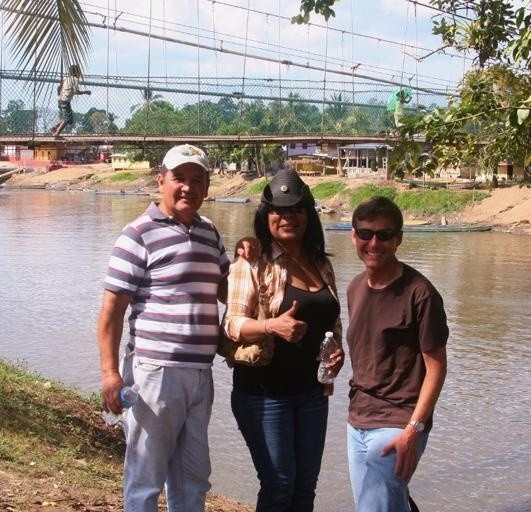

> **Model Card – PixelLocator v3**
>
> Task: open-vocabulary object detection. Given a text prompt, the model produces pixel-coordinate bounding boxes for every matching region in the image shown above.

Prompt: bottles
[104,383,142,427]
[316,331,336,386]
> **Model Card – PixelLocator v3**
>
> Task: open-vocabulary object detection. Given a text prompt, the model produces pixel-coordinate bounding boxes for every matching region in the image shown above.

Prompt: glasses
[354,224,399,241]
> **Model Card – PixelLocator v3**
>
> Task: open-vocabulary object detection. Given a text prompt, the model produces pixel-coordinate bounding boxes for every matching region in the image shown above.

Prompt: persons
[394,91,412,128]
[220,168,347,512]
[344,195,450,511]
[97,145,262,512]
[51,65,92,138]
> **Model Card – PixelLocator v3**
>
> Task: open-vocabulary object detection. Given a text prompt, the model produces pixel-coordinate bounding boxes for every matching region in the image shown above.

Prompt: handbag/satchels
[213,257,272,370]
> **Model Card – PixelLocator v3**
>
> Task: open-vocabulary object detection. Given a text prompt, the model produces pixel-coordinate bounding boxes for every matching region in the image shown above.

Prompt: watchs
[408,420,426,434]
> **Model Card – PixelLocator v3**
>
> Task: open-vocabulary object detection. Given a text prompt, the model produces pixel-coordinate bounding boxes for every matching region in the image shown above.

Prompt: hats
[161,143,210,173]
[261,168,315,209]
